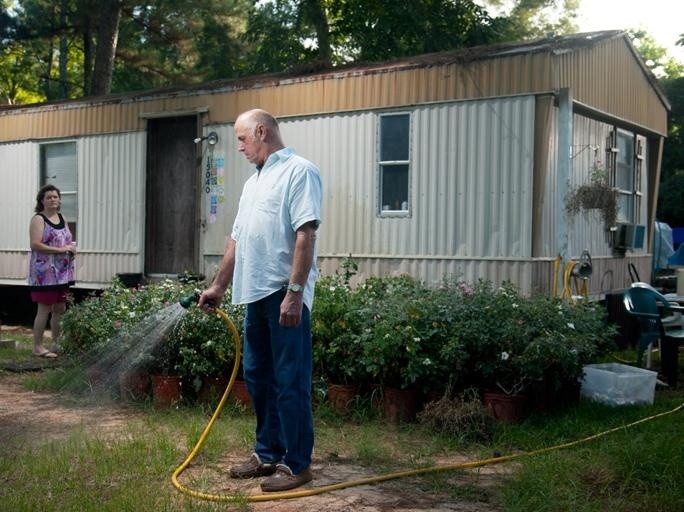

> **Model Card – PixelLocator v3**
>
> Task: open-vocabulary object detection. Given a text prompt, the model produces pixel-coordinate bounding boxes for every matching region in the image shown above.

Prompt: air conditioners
[613,220,646,250]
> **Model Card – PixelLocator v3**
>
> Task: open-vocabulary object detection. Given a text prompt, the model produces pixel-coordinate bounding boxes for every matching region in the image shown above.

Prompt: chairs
[622,281,684,388]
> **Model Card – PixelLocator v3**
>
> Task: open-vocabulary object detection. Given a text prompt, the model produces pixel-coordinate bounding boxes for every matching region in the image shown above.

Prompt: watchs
[281,282,304,295]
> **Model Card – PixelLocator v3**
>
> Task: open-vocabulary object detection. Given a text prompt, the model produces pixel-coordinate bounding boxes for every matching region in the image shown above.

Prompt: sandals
[259,463,313,492]
[230,452,275,479]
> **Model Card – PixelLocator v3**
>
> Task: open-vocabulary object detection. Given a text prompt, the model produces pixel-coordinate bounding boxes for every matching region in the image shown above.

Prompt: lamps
[193,131,218,146]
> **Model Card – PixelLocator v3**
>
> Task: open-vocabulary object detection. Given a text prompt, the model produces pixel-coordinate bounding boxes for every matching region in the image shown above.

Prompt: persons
[26,181,80,361]
[192,107,327,495]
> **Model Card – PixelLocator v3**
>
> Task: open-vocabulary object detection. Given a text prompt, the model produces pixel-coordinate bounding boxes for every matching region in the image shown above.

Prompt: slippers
[32,350,58,358]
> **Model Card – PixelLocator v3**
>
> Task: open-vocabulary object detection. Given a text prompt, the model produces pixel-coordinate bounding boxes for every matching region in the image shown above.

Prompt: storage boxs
[580,363,657,413]
